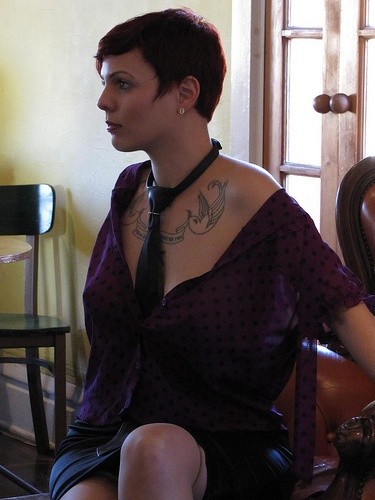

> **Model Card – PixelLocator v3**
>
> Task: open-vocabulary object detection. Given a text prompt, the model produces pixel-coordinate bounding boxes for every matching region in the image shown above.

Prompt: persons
[44,8,374,500]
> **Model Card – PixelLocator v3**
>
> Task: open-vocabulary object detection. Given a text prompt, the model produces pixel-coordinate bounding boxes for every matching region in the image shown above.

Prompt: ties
[125,137,225,342]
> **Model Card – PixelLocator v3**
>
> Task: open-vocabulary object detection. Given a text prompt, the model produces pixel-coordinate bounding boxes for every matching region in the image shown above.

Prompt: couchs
[273,343,375,500]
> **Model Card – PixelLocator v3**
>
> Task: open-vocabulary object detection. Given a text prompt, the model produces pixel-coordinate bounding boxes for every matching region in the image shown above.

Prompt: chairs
[0,183,70,459]
[335,158,375,295]
